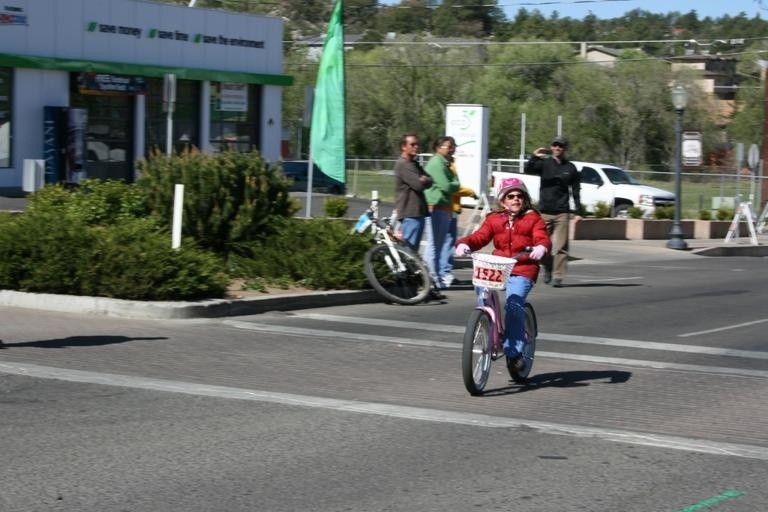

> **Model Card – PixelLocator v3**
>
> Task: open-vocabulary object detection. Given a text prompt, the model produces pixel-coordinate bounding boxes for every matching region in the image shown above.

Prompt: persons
[439,136,479,286]
[523,136,583,287]
[393,131,434,250]
[455,178,552,371]
[424,136,460,288]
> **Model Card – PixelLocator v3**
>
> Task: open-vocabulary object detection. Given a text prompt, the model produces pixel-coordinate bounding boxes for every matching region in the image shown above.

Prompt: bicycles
[461,248,539,396]
[347,196,450,305]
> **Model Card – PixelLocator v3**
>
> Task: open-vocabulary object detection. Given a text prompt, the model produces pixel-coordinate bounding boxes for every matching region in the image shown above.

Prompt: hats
[551,135,567,145]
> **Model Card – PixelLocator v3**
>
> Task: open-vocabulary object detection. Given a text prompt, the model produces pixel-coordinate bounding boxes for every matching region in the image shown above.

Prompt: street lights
[666,82,689,250]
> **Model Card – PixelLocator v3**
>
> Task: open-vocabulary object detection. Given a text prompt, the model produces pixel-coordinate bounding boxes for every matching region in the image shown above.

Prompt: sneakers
[444,275,458,286]
[551,278,561,287]
[542,267,554,282]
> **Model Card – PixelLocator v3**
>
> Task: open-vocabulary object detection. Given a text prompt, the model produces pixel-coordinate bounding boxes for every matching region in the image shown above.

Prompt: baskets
[473,252,521,290]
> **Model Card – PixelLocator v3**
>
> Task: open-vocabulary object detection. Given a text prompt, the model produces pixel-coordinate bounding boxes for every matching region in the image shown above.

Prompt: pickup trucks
[489,155,676,219]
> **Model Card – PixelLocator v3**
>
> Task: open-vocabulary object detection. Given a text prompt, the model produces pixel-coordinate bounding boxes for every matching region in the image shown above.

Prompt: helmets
[497,178,528,199]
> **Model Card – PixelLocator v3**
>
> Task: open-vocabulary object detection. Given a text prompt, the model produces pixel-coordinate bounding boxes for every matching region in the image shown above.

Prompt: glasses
[507,194,524,199]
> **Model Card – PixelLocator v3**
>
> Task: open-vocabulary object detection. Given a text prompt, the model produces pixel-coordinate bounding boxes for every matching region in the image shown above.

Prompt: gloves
[456,244,470,256]
[526,245,545,260]
